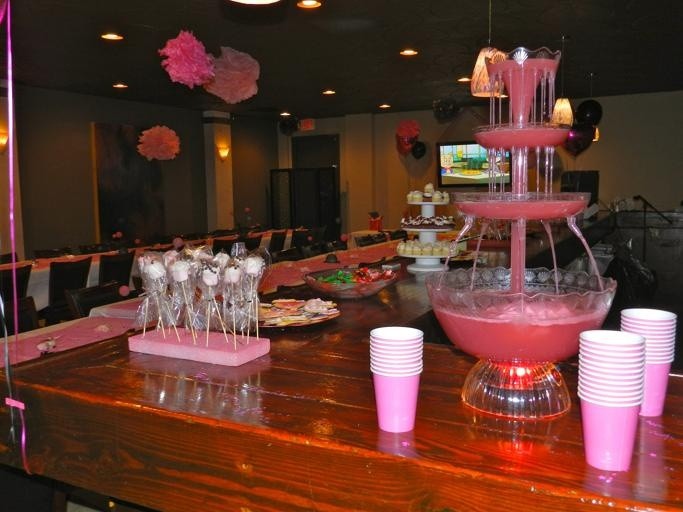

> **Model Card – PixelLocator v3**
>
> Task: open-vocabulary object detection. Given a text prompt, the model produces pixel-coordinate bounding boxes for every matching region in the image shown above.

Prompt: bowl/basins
[304,266,398,298]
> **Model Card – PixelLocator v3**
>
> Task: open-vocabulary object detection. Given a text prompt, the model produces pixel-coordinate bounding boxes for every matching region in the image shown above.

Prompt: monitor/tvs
[435,140,512,188]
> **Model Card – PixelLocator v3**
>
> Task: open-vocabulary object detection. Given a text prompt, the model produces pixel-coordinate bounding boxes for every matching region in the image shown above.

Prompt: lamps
[551,32,599,143]
[471,0,508,98]
[217,146,229,162]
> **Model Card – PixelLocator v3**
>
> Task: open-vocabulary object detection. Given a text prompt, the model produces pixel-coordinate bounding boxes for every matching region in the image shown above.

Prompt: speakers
[560,170,599,220]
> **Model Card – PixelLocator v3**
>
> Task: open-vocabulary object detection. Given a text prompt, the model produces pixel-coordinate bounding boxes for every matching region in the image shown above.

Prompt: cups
[618,307,678,416]
[370,326,424,432]
[577,329,646,471]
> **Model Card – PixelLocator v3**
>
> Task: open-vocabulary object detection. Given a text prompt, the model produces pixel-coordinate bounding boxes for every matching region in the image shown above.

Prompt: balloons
[564,123,594,156]
[276,115,297,137]
[431,96,456,122]
[394,120,425,160]
[575,100,603,127]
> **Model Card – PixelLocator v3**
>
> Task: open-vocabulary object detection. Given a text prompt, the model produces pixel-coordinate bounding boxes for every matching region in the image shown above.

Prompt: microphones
[632,194,647,205]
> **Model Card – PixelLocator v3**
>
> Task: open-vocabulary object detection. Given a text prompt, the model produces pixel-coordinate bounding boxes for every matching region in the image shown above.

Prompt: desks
[0,316,157,369]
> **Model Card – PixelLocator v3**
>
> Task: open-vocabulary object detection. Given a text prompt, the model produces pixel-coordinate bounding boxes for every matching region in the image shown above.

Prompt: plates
[254,299,340,328]
